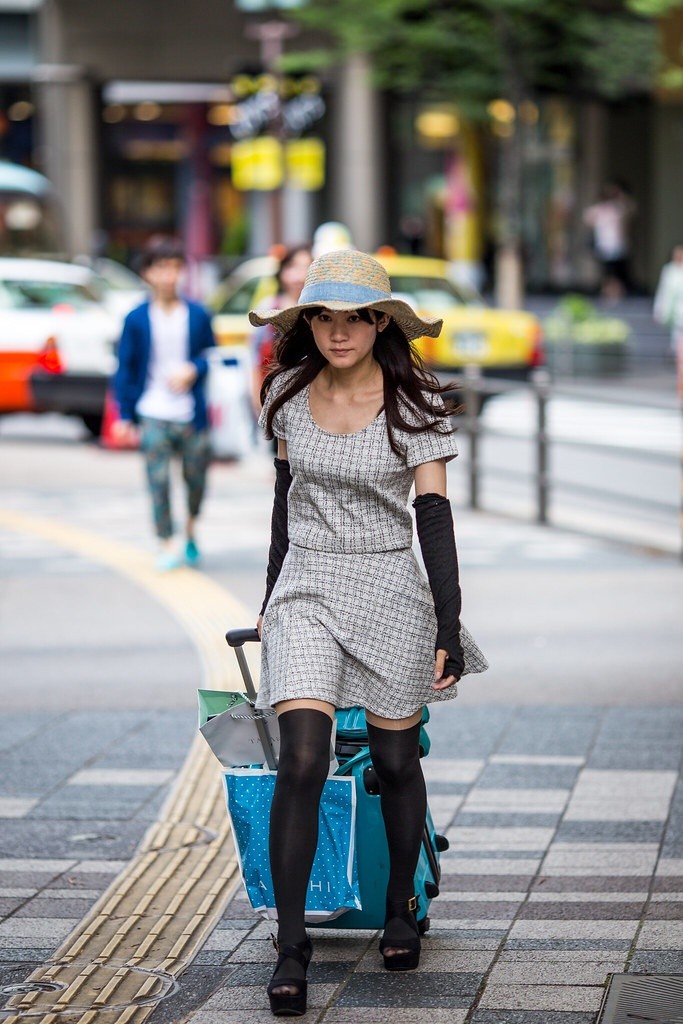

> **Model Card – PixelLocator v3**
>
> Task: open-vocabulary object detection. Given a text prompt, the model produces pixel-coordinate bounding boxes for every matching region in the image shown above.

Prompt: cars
[0,258,121,435]
[205,245,541,374]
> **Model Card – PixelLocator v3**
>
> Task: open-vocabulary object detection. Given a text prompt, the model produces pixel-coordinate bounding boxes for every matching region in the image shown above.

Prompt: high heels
[379,894,421,971]
[267,933,313,1016]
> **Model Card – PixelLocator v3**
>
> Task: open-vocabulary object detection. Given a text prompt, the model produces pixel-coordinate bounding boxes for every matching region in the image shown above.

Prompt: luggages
[226,624,450,934]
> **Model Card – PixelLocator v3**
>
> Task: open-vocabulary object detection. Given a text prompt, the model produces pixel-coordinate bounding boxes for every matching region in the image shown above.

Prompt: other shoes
[160,545,174,569]
[183,532,199,560]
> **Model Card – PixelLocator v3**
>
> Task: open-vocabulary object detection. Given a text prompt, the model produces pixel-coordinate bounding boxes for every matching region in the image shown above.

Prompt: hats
[248,251,444,342]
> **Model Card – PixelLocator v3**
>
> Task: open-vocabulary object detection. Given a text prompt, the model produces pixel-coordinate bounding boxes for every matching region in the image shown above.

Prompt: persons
[576,173,641,299]
[250,250,316,420]
[107,240,222,568]
[244,251,493,1019]
[650,241,683,401]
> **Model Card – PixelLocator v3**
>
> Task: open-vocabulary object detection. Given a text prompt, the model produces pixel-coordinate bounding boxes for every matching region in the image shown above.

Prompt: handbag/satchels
[201,677,301,774]
[224,761,363,924]
